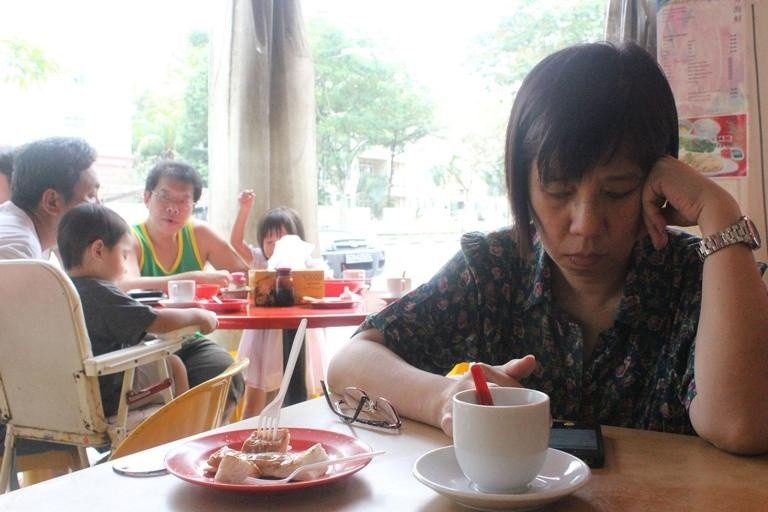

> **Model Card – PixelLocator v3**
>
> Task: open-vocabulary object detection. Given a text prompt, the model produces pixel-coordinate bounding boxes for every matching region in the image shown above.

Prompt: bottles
[228,273,247,290]
[275,268,295,305]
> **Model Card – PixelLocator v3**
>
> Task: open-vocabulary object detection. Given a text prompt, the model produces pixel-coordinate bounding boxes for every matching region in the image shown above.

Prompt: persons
[1,153,12,201]
[121,160,257,428]
[2,136,99,269]
[229,185,310,422]
[56,206,222,419]
[323,40,768,460]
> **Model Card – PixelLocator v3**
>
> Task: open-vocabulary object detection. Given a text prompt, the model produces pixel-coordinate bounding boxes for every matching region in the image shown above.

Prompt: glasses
[319,379,402,430]
[151,190,198,211]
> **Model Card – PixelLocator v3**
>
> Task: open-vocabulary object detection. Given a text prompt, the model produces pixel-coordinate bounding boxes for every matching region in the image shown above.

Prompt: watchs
[695,210,762,262]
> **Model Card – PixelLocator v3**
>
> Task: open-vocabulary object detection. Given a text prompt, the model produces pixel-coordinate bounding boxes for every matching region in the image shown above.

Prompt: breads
[208,429,331,484]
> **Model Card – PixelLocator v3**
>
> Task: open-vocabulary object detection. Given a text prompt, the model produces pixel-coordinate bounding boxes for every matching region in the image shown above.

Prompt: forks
[256,316,308,440]
[244,450,385,485]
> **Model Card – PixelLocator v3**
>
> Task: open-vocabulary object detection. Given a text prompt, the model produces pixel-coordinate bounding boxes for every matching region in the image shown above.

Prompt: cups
[387,278,413,296]
[694,119,721,138]
[220,288,252,304]
[167,280,196,301]
[343,268,365,287]
[452,387,551,492]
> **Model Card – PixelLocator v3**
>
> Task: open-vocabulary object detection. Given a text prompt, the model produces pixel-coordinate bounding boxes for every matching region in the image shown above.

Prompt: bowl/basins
[194,284,220,300]
[323,279,362,297]
[678,119,693,137]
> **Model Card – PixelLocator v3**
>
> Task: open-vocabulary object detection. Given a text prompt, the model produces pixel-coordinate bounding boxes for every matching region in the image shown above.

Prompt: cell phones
[548,421,604,468]
[128,289,163,298]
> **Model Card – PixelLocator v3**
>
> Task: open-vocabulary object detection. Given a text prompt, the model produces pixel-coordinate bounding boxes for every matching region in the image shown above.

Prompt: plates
[381,297,397,303]
[679,139,721,156]
[310,295,353,309]
[410,444,590,512]
[159,299,198,308]
[679,151,739,178]
[162,429,374,492]
[198,299,249,310]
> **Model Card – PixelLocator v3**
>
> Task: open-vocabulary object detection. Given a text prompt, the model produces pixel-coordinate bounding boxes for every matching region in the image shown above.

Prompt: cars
[317,237,388,284]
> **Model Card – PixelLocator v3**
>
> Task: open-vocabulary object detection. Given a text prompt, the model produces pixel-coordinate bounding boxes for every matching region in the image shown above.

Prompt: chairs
[107,356,251,462]
[1,258,206,498]
